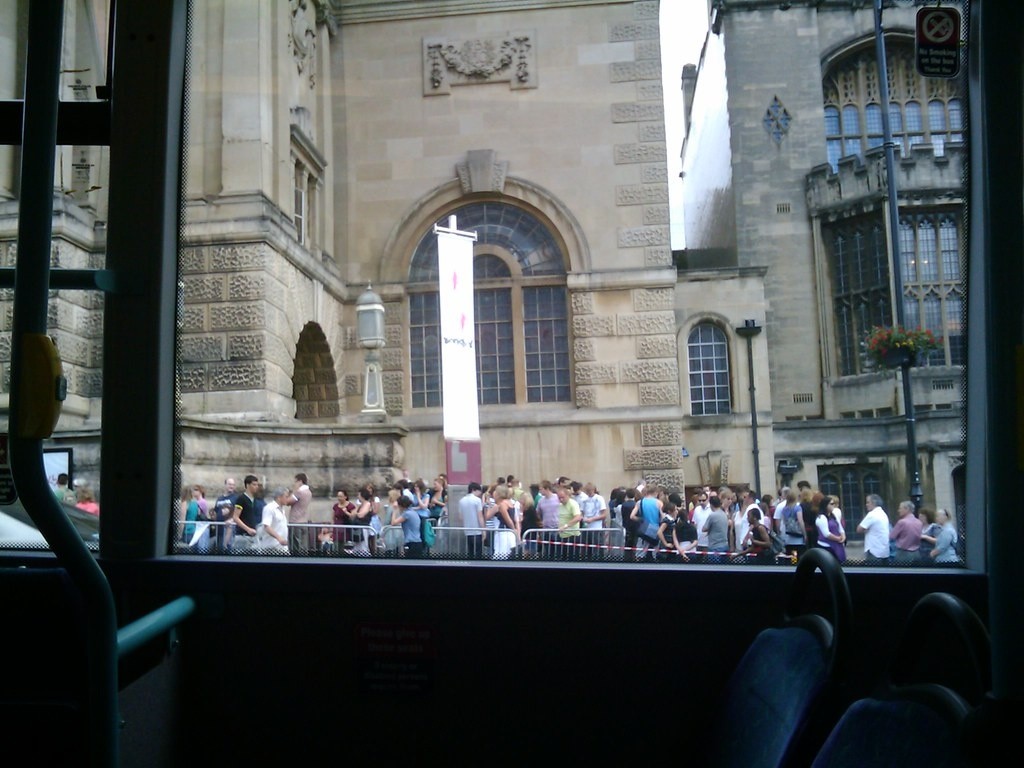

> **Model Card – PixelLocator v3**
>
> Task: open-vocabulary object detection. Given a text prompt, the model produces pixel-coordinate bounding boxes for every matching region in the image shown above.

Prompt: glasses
[698,499,706,502]
[830,503,834,505]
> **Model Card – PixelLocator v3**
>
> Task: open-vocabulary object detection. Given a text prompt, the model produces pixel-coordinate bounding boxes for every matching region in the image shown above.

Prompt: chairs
[812,593,1024,768]
[719,548,855,768]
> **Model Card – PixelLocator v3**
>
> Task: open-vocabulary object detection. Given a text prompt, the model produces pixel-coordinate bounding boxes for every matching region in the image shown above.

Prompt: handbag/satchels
[638,520,659,546]
[419,514,436,547]
[197,505,216,538]
[757,524,785,557]
[349,518,364,542]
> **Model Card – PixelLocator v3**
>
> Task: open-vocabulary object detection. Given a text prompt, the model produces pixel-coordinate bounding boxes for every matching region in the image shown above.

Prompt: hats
[73,478,86,486]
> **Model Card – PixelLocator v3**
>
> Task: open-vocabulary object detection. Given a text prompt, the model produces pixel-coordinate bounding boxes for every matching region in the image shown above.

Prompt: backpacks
[950,532,964,555]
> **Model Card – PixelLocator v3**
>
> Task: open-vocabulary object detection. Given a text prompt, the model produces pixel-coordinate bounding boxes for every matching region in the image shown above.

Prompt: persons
[53,474,99,516]
[174,472,958,563]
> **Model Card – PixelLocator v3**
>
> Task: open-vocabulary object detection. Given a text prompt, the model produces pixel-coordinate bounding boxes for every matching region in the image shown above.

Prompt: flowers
[864,326,944,380]
